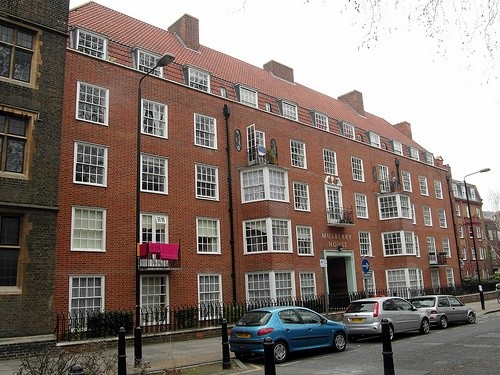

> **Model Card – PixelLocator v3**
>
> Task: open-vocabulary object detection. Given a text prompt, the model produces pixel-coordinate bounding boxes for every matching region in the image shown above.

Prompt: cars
[410,294,477,328]
[342,297,432,343]
[229,307,348,364]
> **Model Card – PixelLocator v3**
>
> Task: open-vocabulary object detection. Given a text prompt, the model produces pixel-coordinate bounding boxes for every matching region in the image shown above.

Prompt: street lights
[133,53,176,366]
[464,167,492,309]
